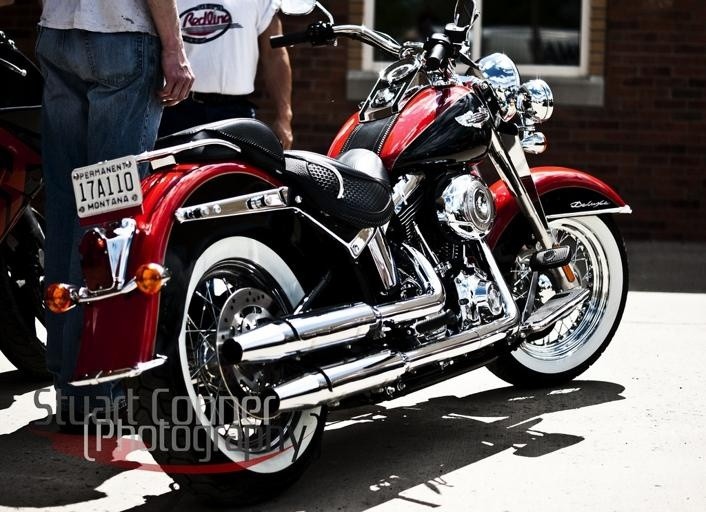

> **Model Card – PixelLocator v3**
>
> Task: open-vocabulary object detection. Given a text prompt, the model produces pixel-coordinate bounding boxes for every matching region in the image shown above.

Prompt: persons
[34,0,195,436]
[153,0,294,153]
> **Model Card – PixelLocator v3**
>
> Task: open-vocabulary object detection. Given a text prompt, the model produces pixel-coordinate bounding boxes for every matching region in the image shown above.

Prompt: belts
[179,91,250,107]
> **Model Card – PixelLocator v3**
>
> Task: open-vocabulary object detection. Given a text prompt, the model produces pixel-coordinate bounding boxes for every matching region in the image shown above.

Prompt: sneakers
[55,390,143,430]
